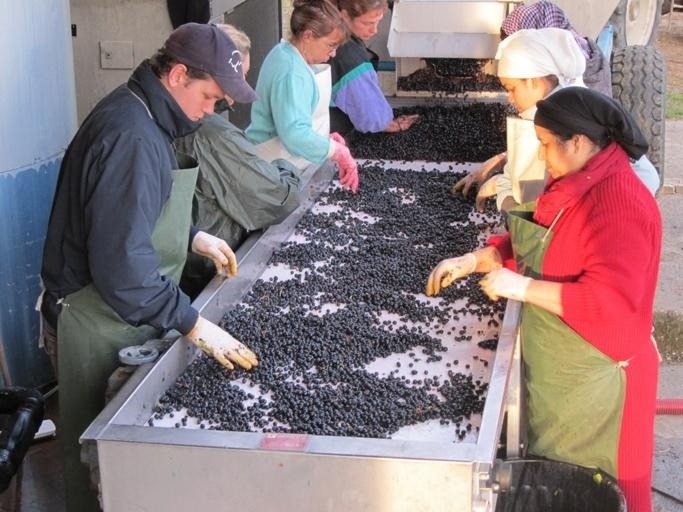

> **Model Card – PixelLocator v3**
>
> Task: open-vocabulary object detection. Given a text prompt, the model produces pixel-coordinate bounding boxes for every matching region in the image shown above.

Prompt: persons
[326,1,419,138]
[32,20,260,511]
[423,86,663,511]
[473,28,660,218]
[451,1,613,199]
[245,1,361,197]
[165,20,303,303]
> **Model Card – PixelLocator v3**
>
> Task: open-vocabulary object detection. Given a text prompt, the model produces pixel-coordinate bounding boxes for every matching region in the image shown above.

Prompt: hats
[158,21,258,105]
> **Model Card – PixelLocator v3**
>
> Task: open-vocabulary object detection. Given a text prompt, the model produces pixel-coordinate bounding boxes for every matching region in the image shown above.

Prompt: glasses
[314,34,341,52]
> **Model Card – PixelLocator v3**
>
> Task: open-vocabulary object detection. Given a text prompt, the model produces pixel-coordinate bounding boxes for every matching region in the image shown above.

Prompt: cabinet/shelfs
[75,94,520,510]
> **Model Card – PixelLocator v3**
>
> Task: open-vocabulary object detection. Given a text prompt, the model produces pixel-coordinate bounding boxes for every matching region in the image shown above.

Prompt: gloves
[422,251,478,297]
[478,265,533,303]
[190,229,238,280]
[451,151,506,197]
[327,130,361,194]
[187,317,259,372]
[475,171,503,212]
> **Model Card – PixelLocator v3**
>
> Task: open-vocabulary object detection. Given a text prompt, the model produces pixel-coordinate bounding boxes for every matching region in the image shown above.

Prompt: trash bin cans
[495,459,627,512]
